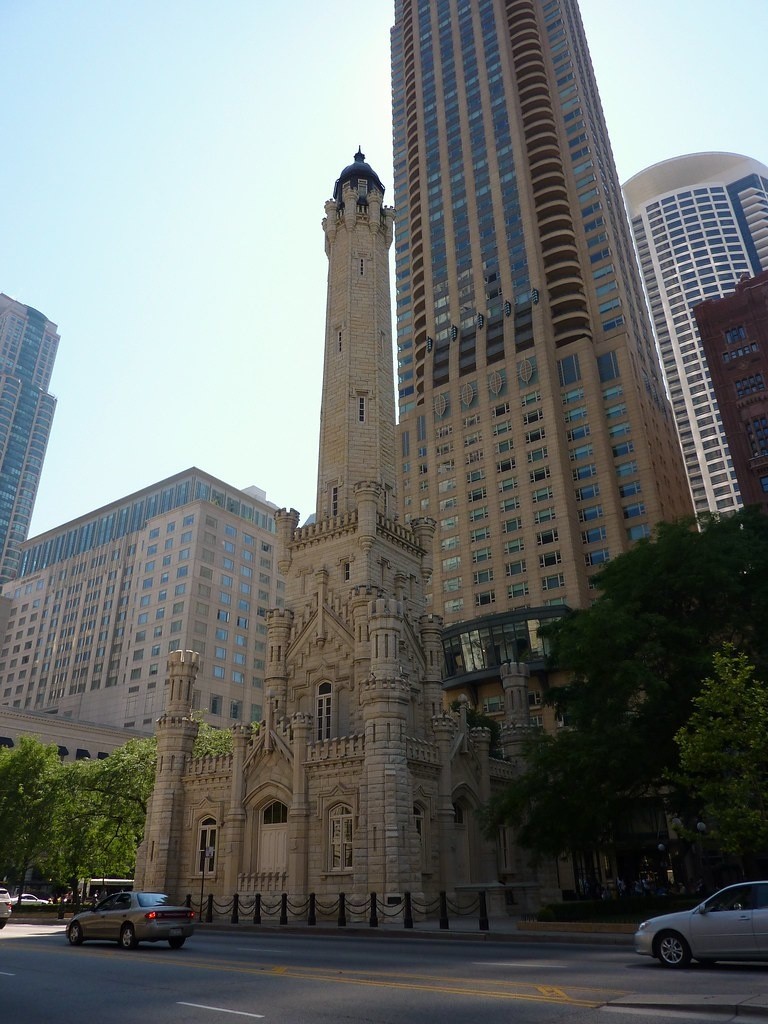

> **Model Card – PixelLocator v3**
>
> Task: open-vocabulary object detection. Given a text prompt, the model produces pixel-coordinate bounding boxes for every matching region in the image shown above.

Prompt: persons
[634,881,640,895]
[99,888,107,899]
[696,878,704,893]
[93,892,98,902]
[498,876,517,905]
[644,873,656,895]
[63,891,73,903]
[79,888,83,898]
[617,877,622,889]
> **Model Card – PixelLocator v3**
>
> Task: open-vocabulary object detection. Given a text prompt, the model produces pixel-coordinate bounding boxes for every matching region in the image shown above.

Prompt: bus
[87,878,134,898]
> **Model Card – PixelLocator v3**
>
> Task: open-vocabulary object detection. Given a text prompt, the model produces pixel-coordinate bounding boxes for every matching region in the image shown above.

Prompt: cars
[634,880,768,969]
[0,887,13,930]
[9,893,50,905]
[65,891,196,951]
